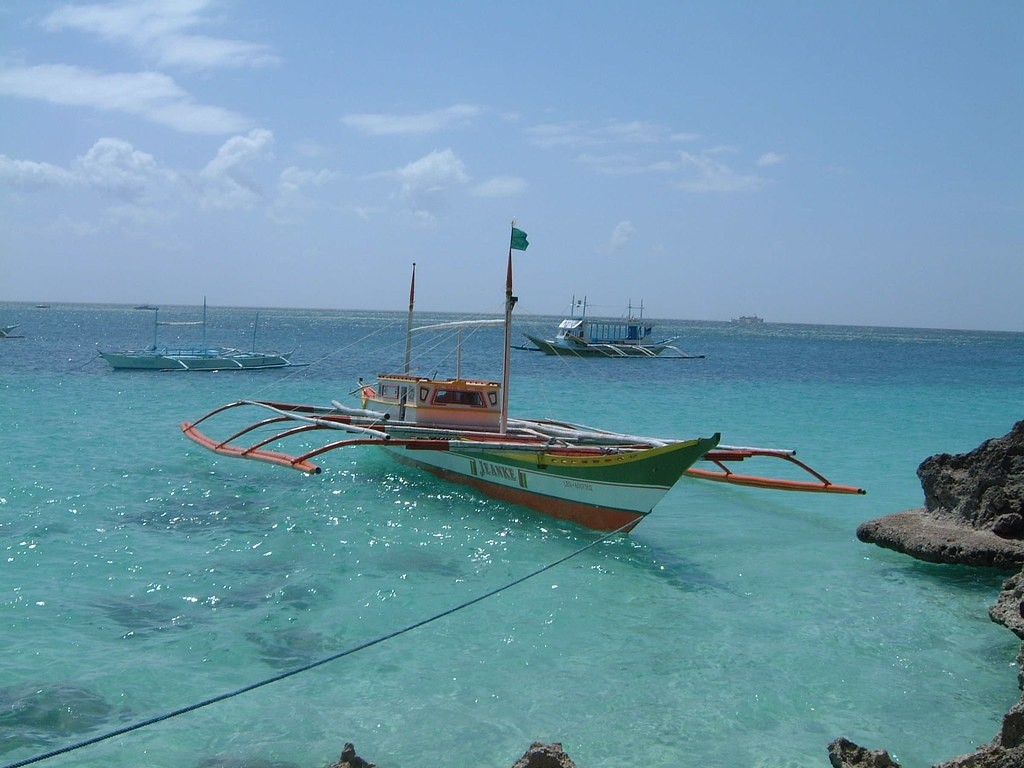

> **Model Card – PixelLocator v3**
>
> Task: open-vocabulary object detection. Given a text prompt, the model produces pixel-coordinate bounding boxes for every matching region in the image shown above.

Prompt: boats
[730,315,765,326]
[131,302,162,310]
[36,303,53,310]
[152,316,206,327]
[523,294,681,355]
[96,295,296,370]
[1,322,26,340]
[371,218,722,538]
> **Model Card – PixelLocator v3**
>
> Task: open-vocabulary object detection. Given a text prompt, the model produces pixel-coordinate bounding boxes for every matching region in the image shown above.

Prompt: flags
[510,228,529,250]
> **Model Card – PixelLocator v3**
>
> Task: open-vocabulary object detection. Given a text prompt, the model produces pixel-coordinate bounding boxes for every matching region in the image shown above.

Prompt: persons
[461,393,475,403]
